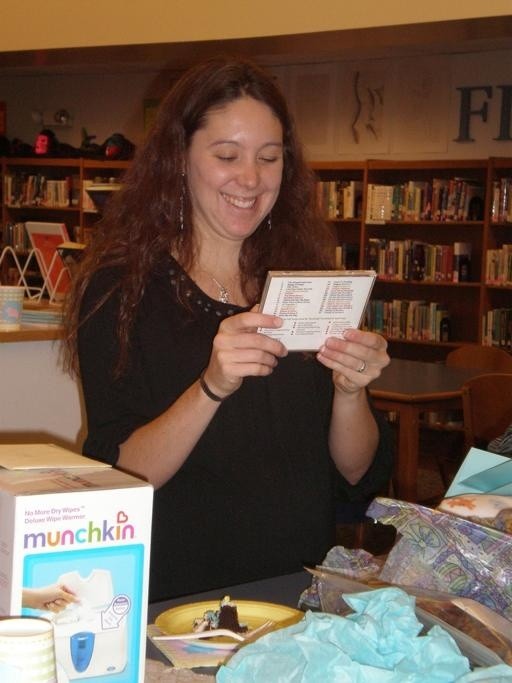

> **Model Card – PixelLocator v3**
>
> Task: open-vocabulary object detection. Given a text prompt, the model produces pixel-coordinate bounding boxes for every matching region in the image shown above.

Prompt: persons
[51,58,391,605]
[21,582,81,612]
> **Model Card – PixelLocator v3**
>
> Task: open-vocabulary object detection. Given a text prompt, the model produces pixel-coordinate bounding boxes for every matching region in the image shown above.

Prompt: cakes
[193,595,247,640]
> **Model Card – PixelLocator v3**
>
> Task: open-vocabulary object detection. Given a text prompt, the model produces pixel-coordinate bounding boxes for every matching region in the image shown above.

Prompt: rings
[356,362,366,373]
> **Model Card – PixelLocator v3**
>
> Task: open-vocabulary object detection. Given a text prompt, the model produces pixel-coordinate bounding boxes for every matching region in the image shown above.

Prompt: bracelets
[200,367,227,402]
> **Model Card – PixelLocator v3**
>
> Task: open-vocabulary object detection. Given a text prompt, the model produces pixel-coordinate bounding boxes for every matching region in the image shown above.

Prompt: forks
[152,618,276,642]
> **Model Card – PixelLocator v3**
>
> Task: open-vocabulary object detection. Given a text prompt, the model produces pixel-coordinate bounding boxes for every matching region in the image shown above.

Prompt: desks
[145,571,321,683]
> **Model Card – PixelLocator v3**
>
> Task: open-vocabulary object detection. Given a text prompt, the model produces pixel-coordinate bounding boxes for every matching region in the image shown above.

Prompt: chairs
[341,345,512,549]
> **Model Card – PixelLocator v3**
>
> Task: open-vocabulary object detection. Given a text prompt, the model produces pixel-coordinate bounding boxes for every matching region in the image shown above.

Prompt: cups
[3,616,55,683]
[0,286,26,331]
[69,629,96,672]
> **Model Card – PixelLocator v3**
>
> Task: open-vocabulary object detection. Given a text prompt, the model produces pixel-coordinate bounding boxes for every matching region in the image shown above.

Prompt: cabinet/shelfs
[0,155,512,364]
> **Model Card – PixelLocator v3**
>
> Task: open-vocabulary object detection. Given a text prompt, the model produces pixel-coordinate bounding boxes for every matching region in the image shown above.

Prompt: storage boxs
[0,442,154,683]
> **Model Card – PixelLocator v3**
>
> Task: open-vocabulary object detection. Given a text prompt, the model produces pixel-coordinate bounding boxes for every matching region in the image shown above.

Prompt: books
[313,177,484,342]
[486,177,511,346]
[1,172,123,301]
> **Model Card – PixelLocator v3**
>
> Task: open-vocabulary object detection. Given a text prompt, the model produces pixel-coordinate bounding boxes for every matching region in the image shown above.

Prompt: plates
[155,597,306,650]
